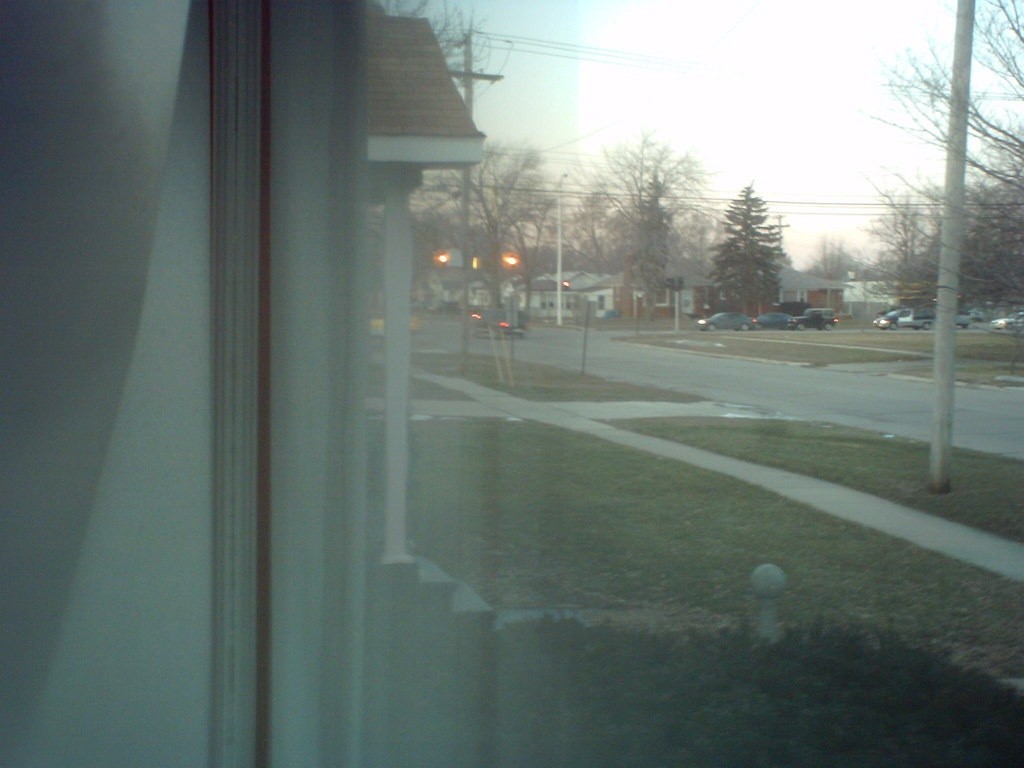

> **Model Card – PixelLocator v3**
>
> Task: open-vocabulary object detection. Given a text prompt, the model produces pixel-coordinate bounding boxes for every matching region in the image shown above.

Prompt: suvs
[794,307,840,330]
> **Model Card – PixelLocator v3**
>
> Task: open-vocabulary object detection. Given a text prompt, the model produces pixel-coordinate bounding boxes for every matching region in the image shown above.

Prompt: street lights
[555,172,565,326]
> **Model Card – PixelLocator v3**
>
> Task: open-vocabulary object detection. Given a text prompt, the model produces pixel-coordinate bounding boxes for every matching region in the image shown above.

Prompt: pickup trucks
[873,308,972,329]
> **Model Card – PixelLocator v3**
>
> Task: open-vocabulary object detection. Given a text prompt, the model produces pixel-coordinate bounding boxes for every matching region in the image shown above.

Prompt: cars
[696,312,757,331]
[756,313,798,331]
[991,311,1024,330]
[596,309,622,320]
